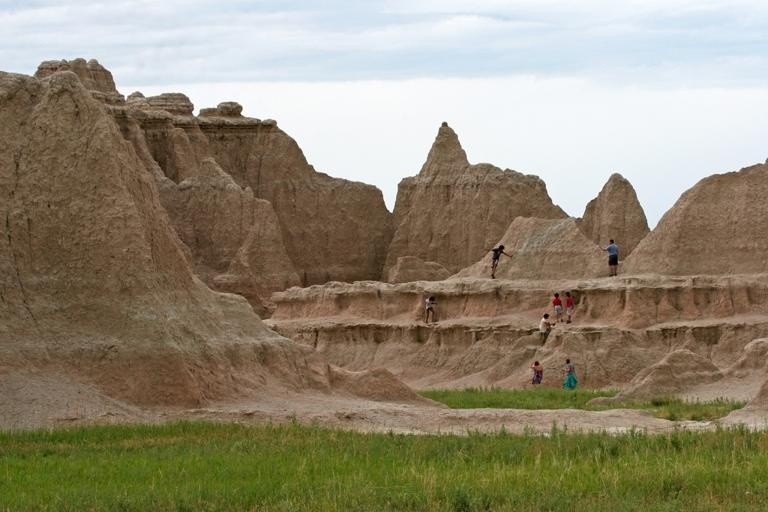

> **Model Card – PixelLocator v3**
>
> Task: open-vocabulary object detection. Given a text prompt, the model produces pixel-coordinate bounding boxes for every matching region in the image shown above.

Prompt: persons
[482,244,512,279]
[530,361,544,385]
[539,313,555,345]
[552,293,563,323]
[565,291,574,324]
[424,295,439,323]
[599,239,619,277]
[561,358,579,391]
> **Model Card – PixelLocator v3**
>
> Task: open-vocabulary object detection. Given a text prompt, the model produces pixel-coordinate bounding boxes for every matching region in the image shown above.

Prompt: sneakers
[609,273,616,276]
